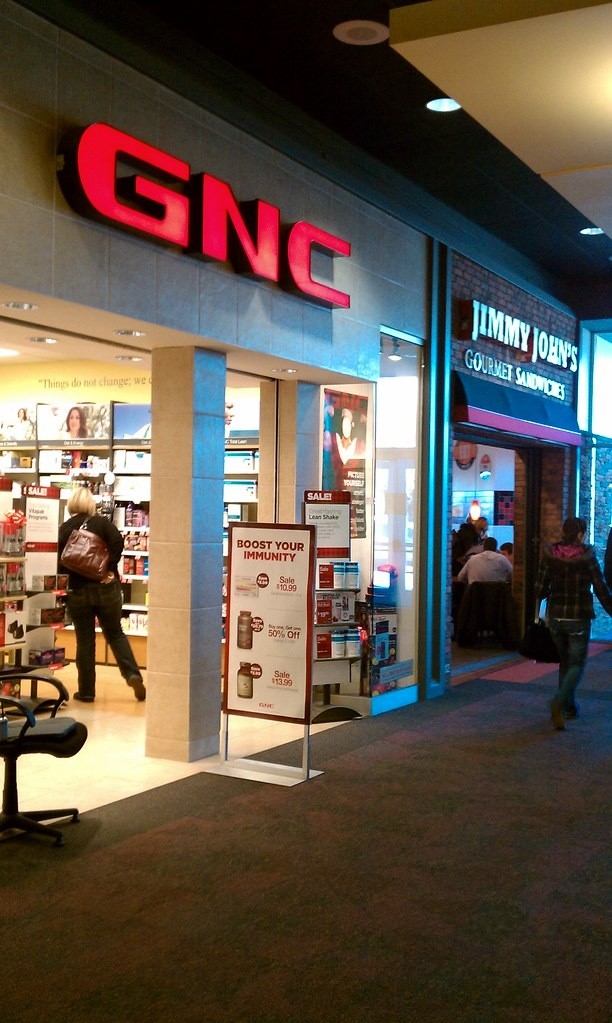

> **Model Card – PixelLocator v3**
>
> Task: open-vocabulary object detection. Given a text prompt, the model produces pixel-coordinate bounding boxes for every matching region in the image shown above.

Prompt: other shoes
[73,691,94,702]
[127,673,146,701]
[549,697,567,730]
[562,687,578,716]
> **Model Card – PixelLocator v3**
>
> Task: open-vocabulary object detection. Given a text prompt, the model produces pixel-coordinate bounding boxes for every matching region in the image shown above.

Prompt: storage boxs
[223,451,254,471]
[224,478,257,499]
[227,504,242,522]
[20,456,33,468]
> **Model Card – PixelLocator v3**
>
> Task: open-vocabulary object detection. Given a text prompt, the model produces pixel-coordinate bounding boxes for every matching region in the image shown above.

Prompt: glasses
[478,526,488,532]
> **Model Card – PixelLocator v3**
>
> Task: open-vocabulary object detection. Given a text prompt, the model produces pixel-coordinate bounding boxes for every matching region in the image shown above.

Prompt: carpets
[480,641,610,684]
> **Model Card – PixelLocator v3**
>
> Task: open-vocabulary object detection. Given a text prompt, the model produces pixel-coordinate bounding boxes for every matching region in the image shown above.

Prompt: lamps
[470,458,481,520]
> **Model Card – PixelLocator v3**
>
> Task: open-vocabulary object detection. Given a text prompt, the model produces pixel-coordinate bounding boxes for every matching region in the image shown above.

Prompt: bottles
[73,480,111,496]
[237,662,253,699]
[237,611,253,650]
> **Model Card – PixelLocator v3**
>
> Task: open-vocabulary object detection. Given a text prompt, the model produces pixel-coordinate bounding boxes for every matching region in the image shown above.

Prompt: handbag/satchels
[518,616,546,659]
[60,515,111,581]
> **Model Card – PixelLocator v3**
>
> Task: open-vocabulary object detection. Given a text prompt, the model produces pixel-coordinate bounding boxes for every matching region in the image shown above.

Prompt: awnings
[450,371,582,448]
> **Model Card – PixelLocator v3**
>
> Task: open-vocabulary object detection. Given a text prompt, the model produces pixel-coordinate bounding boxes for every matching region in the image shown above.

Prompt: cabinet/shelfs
[0,447,259,721]
[309,589,364,724]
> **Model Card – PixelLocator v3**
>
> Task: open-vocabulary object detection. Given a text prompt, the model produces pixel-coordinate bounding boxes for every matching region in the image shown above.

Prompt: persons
[60,406,89,439]
[500,542,513,562]
[458,537,519,650]
[57,486,146,703]
[452,517,489,577]
[604,528,612,594]
[534,518,612,730]
[225,399,235,424]
[10,408,33,440]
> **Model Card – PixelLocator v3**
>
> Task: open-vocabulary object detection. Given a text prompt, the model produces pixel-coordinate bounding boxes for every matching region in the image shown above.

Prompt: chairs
[456,580,516,650]
[0,670,88,848]
